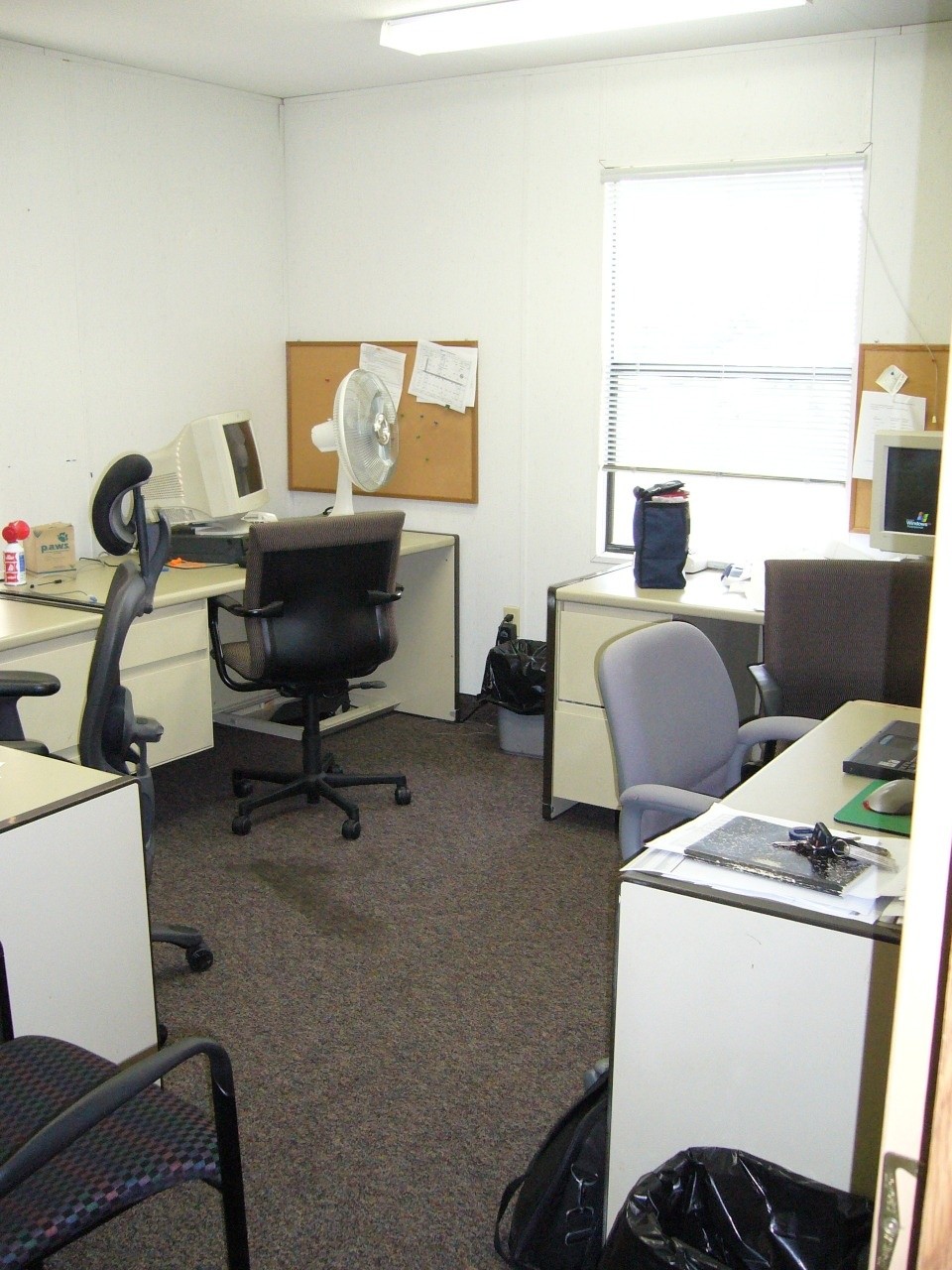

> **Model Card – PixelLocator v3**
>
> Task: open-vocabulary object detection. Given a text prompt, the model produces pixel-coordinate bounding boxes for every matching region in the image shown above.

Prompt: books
[684,815,891,896]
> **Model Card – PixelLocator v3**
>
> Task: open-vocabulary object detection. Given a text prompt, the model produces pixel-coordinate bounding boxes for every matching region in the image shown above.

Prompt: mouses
[862,778,915,816]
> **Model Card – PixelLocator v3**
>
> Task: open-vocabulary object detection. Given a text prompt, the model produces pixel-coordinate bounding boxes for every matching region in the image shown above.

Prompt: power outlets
[504,606,520,637]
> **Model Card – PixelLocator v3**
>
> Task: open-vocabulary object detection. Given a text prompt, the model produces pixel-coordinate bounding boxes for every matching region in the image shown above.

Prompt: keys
[772,823,861,856]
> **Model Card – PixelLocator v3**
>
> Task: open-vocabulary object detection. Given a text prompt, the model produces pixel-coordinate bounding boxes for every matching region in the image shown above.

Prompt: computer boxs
[169,523,252,565]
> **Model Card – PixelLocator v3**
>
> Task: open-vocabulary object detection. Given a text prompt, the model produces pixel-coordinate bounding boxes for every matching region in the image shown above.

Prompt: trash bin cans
[625,1148,871,1270]
[491,639,547,757]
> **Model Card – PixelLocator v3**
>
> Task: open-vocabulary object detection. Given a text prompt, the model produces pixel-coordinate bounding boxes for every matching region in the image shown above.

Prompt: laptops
[841,719,920,779]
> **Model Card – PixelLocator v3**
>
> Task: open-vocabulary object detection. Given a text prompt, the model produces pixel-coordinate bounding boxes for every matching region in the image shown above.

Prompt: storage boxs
[23,521,77,573]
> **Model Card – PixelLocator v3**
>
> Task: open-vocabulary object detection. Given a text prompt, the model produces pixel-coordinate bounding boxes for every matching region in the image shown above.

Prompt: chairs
[746,560,932,773]
[206,510,414,841]
[0,454,215,1050]
[0,940,251,1270]
[593,621,826,860]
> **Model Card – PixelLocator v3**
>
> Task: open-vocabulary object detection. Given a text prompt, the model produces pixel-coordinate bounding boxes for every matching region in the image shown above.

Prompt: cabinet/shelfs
[543,561,764,820]
[600,700,921,1244]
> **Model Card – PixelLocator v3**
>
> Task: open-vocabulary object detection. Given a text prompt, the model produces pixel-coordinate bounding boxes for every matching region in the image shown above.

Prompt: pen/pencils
[30,580,64,588]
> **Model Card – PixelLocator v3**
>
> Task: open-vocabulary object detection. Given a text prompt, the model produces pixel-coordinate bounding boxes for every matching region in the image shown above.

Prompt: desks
[0,747,164,1089]
[0,530,461,773]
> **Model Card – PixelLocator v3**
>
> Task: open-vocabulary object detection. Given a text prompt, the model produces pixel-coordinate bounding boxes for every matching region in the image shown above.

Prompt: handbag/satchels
[494,1069,609,1270]
[632,481,690,589]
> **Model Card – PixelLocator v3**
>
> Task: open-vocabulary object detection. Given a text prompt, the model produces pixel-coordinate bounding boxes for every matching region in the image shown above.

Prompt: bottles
[652,489,689,500]
[2,541,26,585]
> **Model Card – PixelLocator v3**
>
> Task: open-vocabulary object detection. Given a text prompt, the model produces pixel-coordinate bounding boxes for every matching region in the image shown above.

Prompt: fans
[310,368,400,517]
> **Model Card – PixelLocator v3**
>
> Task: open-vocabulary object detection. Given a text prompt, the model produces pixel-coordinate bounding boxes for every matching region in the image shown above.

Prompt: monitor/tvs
[129,409,269,536]
[869,430,944,555]
